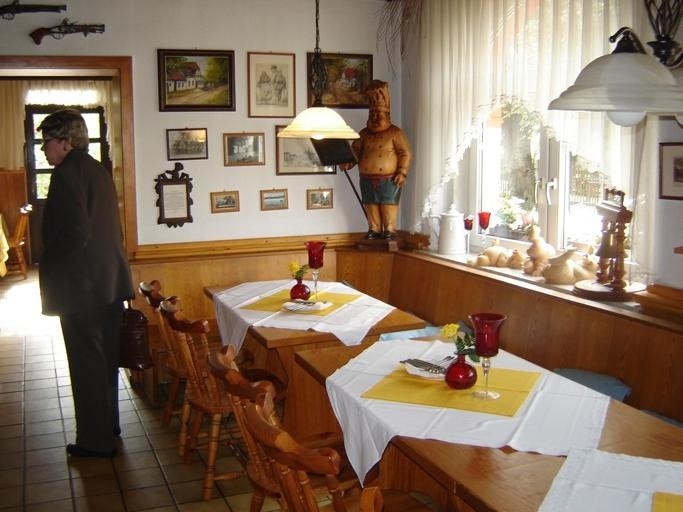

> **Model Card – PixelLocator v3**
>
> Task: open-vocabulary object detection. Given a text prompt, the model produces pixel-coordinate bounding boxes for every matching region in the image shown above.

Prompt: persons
[38,109,135,459]
[327,63,340,103]
[338,79,410,240]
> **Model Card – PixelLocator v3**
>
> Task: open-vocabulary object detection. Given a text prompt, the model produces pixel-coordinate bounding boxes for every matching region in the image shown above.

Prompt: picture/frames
[258,188,288,211]
[305,51,374,110]
[210,189,240,214]
[222,131,266,167]
[305,188,334,211]
[164,127,209,161]
[246,49,298,120]
[657,138,682,202]
[274,123,337,176]
[155,46,237,114]
[152,162,193,228]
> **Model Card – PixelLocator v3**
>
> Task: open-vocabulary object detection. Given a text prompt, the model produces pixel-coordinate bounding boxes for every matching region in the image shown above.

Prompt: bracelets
[398,172,407,178]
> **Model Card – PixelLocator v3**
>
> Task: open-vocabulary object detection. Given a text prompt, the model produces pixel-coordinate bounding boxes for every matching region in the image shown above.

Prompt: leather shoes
[66,440,119,460]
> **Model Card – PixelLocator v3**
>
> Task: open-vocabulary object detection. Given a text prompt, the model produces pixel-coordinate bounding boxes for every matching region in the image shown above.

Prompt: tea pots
[429,203,466,256]
[542,247,577,285]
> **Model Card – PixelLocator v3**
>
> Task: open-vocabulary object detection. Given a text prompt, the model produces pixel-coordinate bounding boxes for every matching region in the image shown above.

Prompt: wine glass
[304,241,328,306]
[469,311,509,402]
[463,218,474,257]
[478,211,491,254]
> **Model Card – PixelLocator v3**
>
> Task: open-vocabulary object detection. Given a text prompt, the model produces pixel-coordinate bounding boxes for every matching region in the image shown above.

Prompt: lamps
[274,0,364,141]
[545,0,683,131]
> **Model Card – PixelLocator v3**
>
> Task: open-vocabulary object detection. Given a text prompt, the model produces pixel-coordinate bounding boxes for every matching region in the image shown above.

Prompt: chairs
[139,280,247,502]
[4,202,33,281]
[207,343,430,512]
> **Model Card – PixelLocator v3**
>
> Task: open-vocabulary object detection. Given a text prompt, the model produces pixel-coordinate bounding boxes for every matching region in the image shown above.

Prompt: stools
[643,407,679,430]
[554,368,630,401]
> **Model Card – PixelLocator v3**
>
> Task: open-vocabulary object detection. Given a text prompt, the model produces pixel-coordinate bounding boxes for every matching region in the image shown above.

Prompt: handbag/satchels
[118,308,154,371]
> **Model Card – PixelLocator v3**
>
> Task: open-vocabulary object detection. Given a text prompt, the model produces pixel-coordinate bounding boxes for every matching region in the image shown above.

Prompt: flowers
[289,259,310,279]
